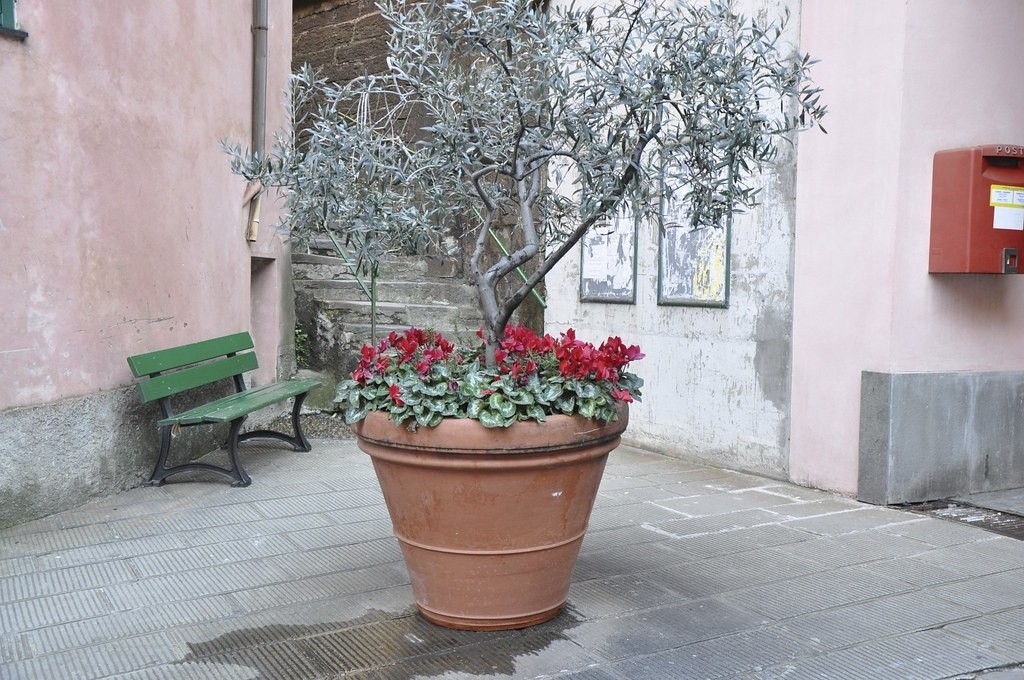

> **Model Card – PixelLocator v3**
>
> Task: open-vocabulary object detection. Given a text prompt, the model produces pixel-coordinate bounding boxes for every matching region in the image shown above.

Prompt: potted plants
[229,0,829,634]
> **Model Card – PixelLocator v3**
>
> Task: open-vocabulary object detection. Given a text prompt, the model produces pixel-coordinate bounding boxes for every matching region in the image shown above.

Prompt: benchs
[126,330,323,489]
